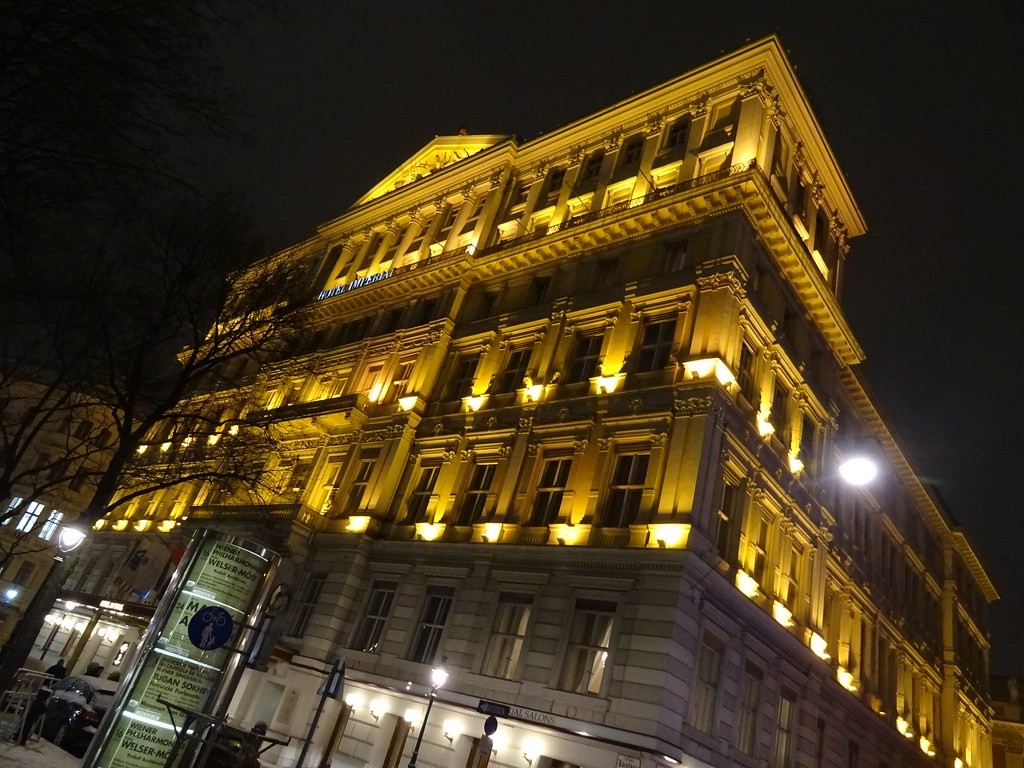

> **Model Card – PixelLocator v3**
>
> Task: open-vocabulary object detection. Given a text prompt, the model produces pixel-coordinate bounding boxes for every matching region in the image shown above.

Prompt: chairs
[0,667,60,746]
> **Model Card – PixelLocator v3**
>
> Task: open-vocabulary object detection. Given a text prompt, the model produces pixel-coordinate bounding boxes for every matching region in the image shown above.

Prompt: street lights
[0,507,96,667]
[405,655,452,768]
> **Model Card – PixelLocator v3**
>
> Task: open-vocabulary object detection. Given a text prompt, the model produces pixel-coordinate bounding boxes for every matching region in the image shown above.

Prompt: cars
[22,674,119,758]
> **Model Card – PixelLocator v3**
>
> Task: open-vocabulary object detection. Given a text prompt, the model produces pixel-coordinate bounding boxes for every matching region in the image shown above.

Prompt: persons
[43,658,67,690]
